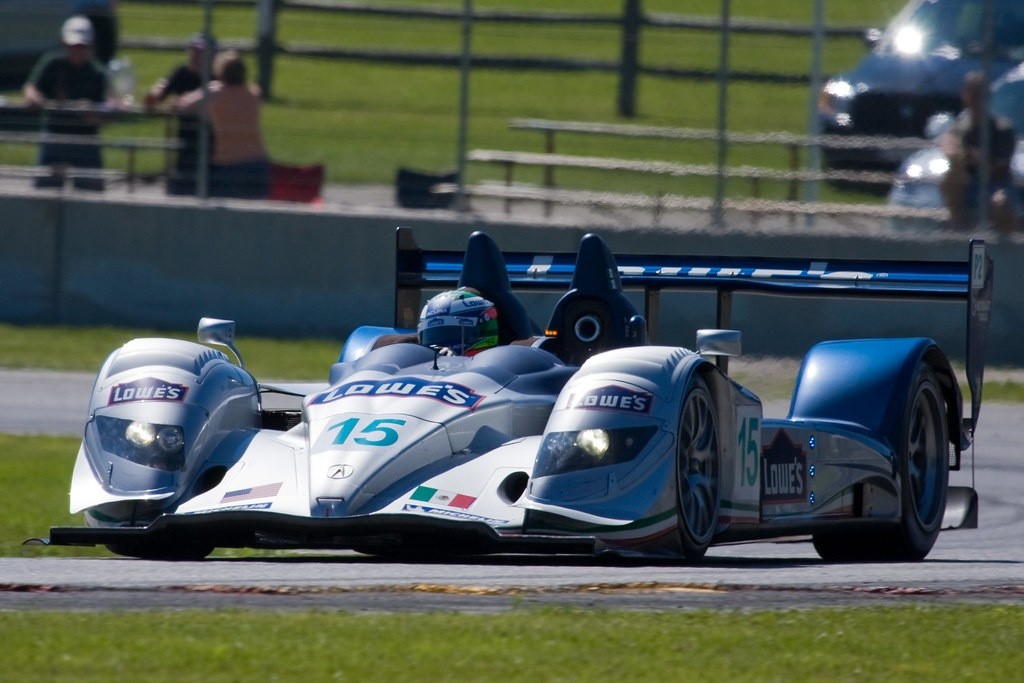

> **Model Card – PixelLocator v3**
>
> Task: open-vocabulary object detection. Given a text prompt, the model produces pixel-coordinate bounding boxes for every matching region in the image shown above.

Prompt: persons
[27,16,125,194]
[141,30,273,198]
[936,74,1018,222]
[419,288,497,357]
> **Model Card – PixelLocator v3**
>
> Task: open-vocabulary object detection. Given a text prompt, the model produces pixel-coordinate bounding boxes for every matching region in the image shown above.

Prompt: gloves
[430,345,457,357]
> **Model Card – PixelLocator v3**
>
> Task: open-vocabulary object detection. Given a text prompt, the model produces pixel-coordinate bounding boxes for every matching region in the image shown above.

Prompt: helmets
[417,289,499,356]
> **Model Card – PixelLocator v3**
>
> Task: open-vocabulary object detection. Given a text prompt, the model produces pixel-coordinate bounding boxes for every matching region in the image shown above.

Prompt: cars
[888,63,1024,228]
[814,1,1022,200]
[1,0,120,89]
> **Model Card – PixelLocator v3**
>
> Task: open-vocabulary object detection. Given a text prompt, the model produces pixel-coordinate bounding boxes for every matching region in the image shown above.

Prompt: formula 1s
[46,218,994,570]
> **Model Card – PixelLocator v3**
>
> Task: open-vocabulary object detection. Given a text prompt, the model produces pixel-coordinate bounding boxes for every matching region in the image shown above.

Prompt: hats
[191,33,218,52]
[61,16,94,46]
